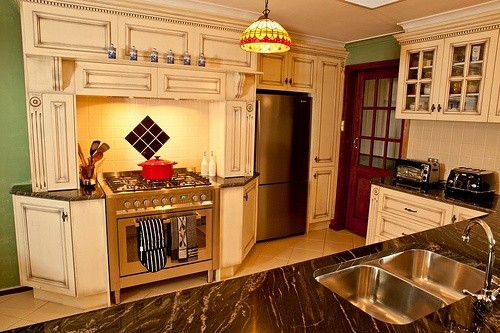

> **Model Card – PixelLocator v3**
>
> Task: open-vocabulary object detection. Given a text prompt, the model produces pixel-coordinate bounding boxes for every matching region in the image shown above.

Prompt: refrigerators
[254,93,313,240]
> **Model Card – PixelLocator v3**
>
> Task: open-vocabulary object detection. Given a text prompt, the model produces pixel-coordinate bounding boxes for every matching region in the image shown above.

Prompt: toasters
[446,166,495,202]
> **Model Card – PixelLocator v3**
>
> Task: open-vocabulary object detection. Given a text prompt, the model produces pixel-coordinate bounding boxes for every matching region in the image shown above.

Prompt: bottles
[150,48,158,64]
[167,49,174,64]
[108,44,116,60]
[198,52,205,68]
[200,151,208,175]
[129,45,137,62]
[183,51,191,66]
[208,150,217,177]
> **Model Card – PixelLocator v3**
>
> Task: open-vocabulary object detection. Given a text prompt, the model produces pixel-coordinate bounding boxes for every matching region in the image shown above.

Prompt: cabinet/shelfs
[395,28,500,124]
[365,184,489,246]
[12,1,351,311]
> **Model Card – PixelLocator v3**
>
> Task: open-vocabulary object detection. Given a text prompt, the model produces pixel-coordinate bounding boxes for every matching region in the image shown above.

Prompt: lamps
[240,0,292,54]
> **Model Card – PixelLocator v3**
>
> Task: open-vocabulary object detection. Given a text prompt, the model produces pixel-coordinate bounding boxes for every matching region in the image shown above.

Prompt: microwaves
[393,159,440,190]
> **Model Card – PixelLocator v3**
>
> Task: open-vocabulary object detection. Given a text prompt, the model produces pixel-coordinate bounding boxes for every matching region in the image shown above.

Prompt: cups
[80,164,97,189]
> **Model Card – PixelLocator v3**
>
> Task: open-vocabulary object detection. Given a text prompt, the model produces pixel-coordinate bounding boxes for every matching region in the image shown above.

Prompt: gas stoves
[100,166,215,218]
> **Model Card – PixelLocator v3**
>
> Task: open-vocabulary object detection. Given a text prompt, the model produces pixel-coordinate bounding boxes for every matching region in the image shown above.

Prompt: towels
[136,218,168,273]
[169,215,198,263]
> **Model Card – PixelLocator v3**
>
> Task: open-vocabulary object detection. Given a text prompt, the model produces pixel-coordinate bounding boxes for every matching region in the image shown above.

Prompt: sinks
[312,259,448,325]
[378,248,496,306]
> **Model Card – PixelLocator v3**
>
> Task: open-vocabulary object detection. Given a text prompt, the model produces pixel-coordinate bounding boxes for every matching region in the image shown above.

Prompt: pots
[136,155,177,183]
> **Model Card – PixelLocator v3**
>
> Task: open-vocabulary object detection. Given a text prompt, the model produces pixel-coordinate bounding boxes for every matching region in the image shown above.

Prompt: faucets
[460,217,496,311]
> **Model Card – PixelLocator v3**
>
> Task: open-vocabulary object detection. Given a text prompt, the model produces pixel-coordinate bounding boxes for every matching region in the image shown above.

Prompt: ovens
[107,206,217,305]
